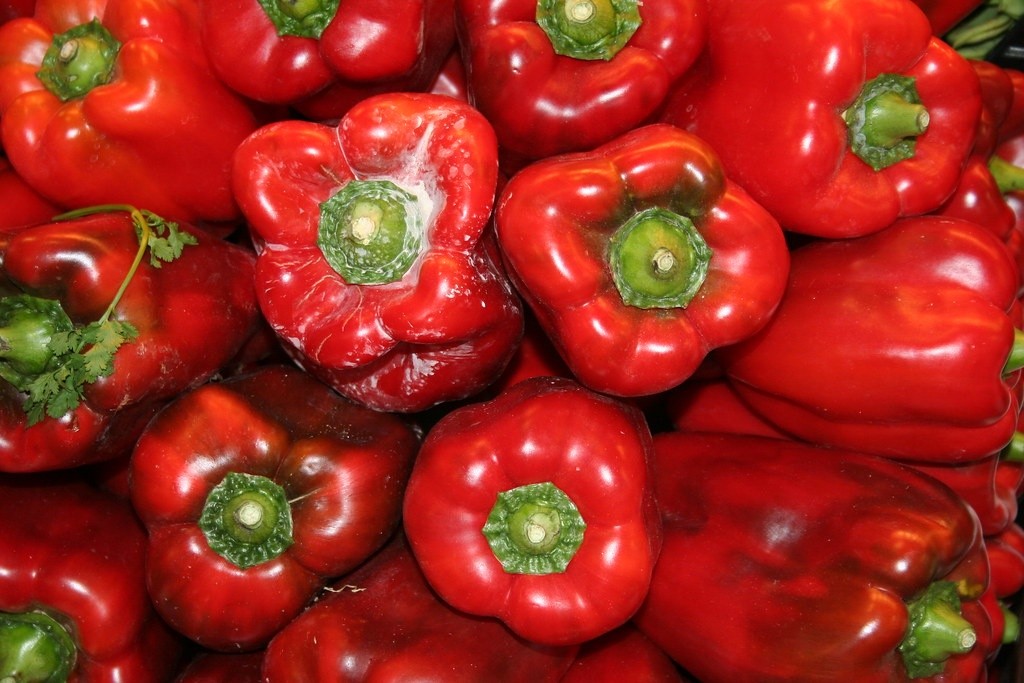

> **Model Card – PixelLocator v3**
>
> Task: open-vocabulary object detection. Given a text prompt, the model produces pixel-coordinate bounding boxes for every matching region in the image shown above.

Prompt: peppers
[1,1,1024,683]
